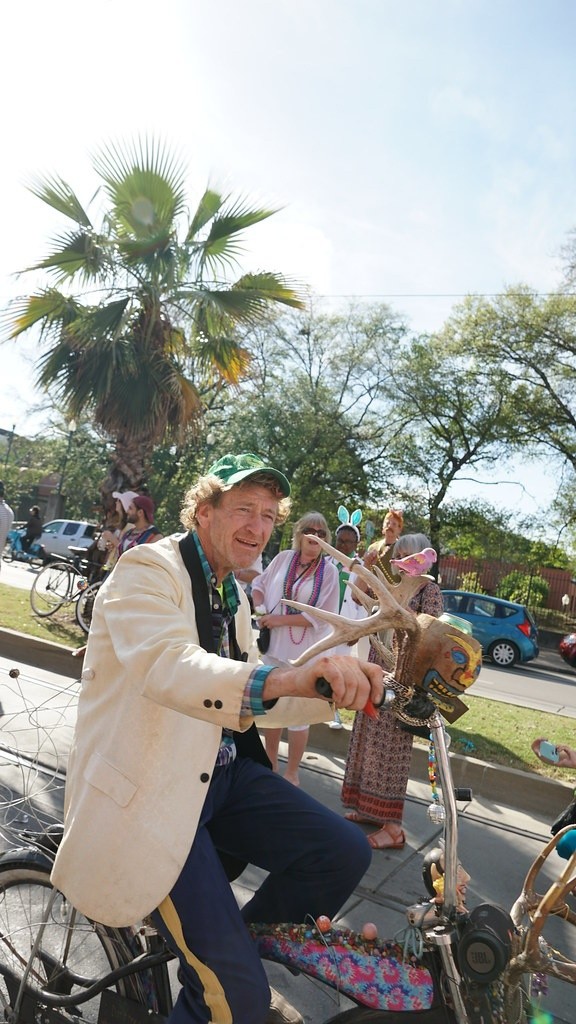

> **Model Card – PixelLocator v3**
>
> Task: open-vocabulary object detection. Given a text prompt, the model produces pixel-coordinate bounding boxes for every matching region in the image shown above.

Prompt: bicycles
[29,546,104,635]
[0,527,46,570]
[0,661,576,1024]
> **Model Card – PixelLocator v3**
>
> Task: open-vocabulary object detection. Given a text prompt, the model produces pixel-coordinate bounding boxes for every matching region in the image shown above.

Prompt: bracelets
[237,569,242,579]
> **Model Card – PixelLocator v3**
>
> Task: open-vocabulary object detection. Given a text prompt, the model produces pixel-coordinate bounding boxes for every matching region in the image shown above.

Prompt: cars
[437,589,541,671]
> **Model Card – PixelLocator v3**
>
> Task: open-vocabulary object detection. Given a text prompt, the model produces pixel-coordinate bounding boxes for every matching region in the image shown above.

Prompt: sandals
[366,825,405,849]
[344,811,384,827]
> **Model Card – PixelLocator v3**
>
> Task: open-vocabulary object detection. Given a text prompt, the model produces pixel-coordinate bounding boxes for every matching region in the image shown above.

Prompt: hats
[208,453,290,498]
[134,496,155,522]
[112,490,139,512]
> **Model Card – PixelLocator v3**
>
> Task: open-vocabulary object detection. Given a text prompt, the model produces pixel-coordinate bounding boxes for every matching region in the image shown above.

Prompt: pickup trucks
[19,518,102,575]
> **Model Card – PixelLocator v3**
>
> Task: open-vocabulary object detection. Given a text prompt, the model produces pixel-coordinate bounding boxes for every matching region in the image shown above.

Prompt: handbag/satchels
[256,625,271,653]
[86,533,108,563]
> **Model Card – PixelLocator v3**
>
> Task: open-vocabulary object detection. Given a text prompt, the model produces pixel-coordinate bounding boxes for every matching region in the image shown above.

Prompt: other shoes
[268,985,305,1024]
[329,710,342,729]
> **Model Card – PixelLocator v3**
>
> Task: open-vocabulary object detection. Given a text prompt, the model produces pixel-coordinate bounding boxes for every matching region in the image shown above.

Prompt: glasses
[337,540,359,546]
[303,527,327,538]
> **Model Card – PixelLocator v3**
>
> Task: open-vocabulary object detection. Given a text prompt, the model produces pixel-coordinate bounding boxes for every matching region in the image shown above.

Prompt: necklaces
[284,551,326,647]
[377,544,397,586]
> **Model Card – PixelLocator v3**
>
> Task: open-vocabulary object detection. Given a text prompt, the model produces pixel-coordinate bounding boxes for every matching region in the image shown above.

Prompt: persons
[94,490,164,586]
[233,552,263,594]
[250,512,340,787]
[361,510,407,651]
[326,507,364,609]
[0,479,14,554]
[17,505,42,552]
[50,455,383,1024]
[341,534,444,848]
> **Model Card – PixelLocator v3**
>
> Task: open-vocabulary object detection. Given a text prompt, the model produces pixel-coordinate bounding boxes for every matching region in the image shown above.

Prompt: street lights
[200,433,216,477]
[3,416,19,467]
[51,418,78,520]
